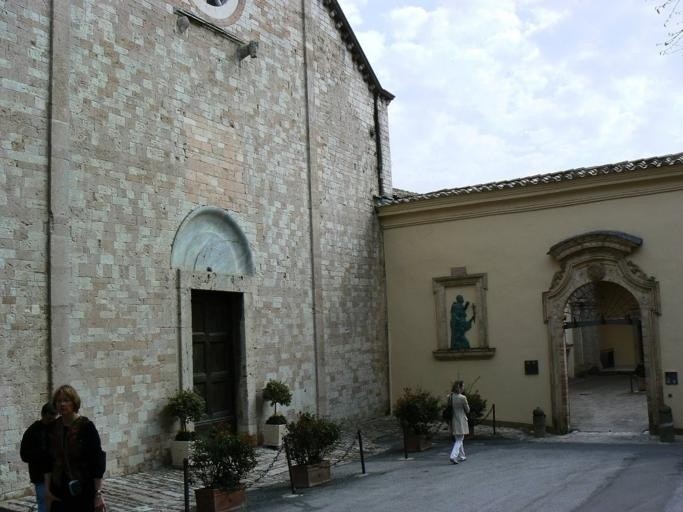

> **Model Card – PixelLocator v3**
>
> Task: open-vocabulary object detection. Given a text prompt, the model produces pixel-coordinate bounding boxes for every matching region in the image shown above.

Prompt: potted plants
[393,384,445,452]
[186,424,260,512]
[453,376,489,440]
[168,390,207,468]
[262,378,292,449]
[284,410,339,489]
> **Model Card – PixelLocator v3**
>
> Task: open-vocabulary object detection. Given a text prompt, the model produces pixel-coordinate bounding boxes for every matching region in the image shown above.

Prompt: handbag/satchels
[443,406,453,420]
[68,480,83,497]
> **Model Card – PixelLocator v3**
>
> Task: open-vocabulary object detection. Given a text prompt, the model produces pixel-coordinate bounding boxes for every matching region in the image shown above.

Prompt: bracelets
[95,487,103,496]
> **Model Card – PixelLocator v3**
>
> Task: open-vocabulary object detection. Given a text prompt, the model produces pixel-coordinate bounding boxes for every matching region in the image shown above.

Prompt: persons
[42,384,108,512]
[446,379,471,465]
[19,402,57,512]
[450,294,473,349]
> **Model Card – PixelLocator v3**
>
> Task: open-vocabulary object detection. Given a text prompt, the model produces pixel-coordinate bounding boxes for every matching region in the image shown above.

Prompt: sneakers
[460,456,466,461]
[450,456,459,464]
